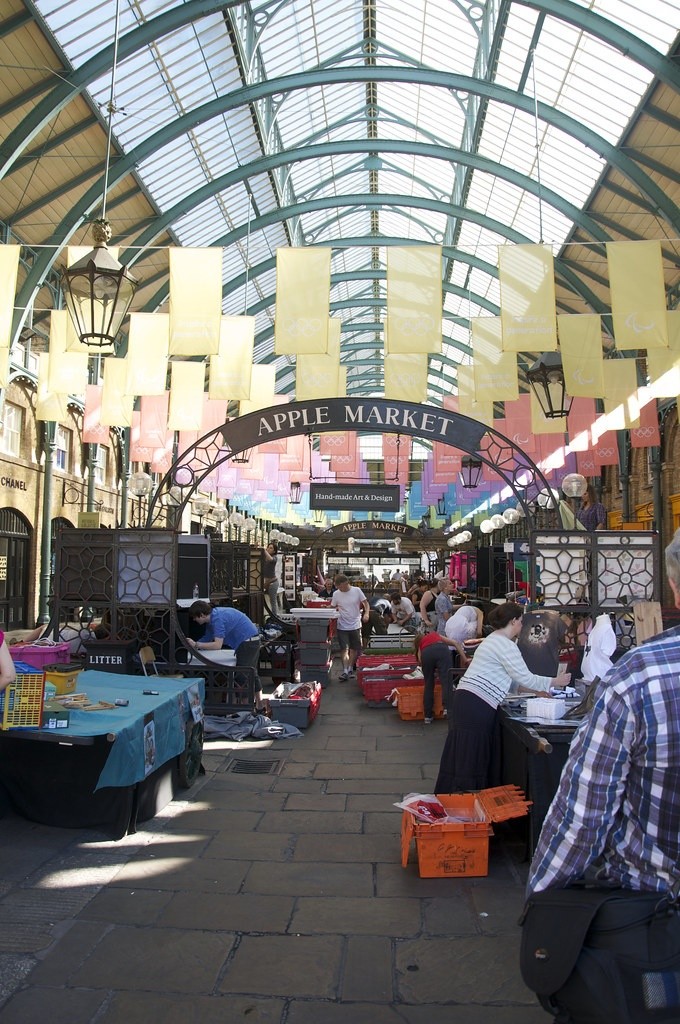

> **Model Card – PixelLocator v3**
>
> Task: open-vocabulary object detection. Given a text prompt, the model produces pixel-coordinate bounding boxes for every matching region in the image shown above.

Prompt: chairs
[138,647,183,678]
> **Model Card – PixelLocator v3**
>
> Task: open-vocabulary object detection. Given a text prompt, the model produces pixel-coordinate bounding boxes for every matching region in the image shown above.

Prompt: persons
[185,600,263,707]
[434,580,454,637]
[445,603,485,689]
[433,602,571,794]
[319,579,335,598]
[525,526,680,1024]
[420,578,442,635]
[381,569,430,612]
[362,593,391,647]
[320,576,369,680]
[0,629,16,692]
[262,543,280,614]
[391,593,416,632]
[418,633,472,724]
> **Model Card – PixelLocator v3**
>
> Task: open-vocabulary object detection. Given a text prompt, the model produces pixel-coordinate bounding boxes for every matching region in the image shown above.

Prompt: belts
[244,636,260,641]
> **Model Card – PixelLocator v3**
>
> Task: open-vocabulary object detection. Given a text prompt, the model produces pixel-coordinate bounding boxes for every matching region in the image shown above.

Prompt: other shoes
[348,670,355,677]
[425,717,433,724]
[339,673,349,680]
[442,708,447,719]
[254,708,265,716]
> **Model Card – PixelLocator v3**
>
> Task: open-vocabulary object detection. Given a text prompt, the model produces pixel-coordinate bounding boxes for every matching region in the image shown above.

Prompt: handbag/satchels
[518,889,680,1024]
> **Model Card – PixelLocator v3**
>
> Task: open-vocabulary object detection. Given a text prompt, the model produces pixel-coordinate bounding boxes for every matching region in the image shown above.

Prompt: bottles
[193,586,198,599]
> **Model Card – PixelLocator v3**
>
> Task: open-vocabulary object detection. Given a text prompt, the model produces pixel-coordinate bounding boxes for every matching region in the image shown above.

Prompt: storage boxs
[268,618,333,728]
[356,654,443,720]
[528,698,568,719]
[401,784,533,878]
[0,642,84,731]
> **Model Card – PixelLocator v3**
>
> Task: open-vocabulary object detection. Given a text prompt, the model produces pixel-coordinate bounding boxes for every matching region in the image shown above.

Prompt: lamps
[228,193,253,464]
[131,468,302,550]
[313,509,323,522]
[57,0,145,345]
[457,453,483,489]
[422,509,433,529]
[525,47,575,419]
[447,473,594,547]
[435,493,447,515]
